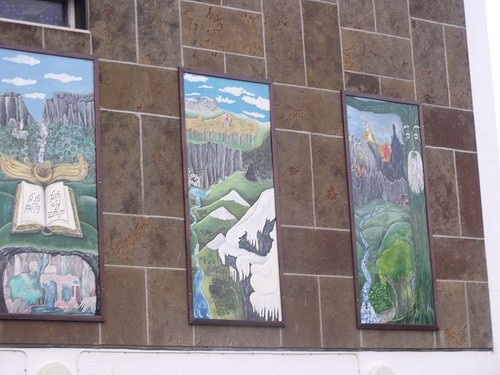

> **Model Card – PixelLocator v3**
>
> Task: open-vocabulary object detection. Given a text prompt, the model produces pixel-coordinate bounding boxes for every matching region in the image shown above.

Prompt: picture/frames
[340,89,440,332]
[177,66,286,329]
[0,43,107,323]
